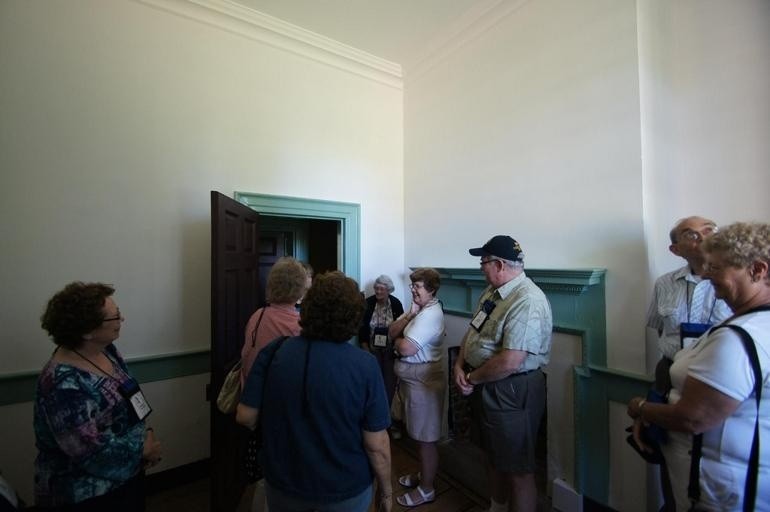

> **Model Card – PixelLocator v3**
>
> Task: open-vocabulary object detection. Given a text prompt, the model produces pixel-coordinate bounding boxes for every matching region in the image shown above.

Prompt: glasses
[480,259,506,265]
[408,283,420,289]
[104,307,121,321]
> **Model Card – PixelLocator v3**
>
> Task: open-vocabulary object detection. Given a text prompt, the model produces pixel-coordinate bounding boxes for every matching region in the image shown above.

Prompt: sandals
[396,471,435,507]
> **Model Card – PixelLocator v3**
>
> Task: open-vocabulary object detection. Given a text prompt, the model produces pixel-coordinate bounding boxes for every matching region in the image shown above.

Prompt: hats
[469,235,524,261]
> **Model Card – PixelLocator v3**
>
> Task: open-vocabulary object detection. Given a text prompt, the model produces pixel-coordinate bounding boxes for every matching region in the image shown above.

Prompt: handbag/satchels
[216,360,241,414]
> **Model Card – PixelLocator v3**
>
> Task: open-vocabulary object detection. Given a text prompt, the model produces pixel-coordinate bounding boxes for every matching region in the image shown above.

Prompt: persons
[33,279,155,512]
[454,235,554,508]
[388,267,447,506]
[357,273,406,439]
